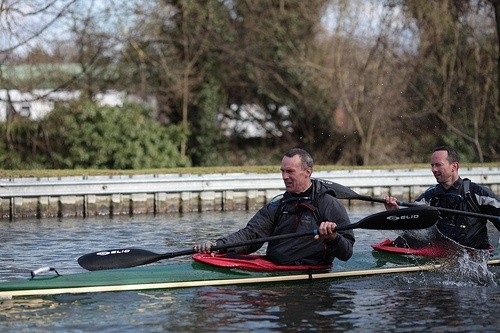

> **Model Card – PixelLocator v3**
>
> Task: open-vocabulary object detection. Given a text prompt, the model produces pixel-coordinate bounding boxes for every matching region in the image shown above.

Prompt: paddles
[78,207,442,271]
[321,180,500,222]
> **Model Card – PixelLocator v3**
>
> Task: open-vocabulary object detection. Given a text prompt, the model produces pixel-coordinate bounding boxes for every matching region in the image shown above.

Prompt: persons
[192,148,355,266]
[383,145,500,250]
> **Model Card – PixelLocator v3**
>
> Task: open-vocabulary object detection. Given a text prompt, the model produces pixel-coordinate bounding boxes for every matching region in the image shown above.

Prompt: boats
[0,243,500,299]
